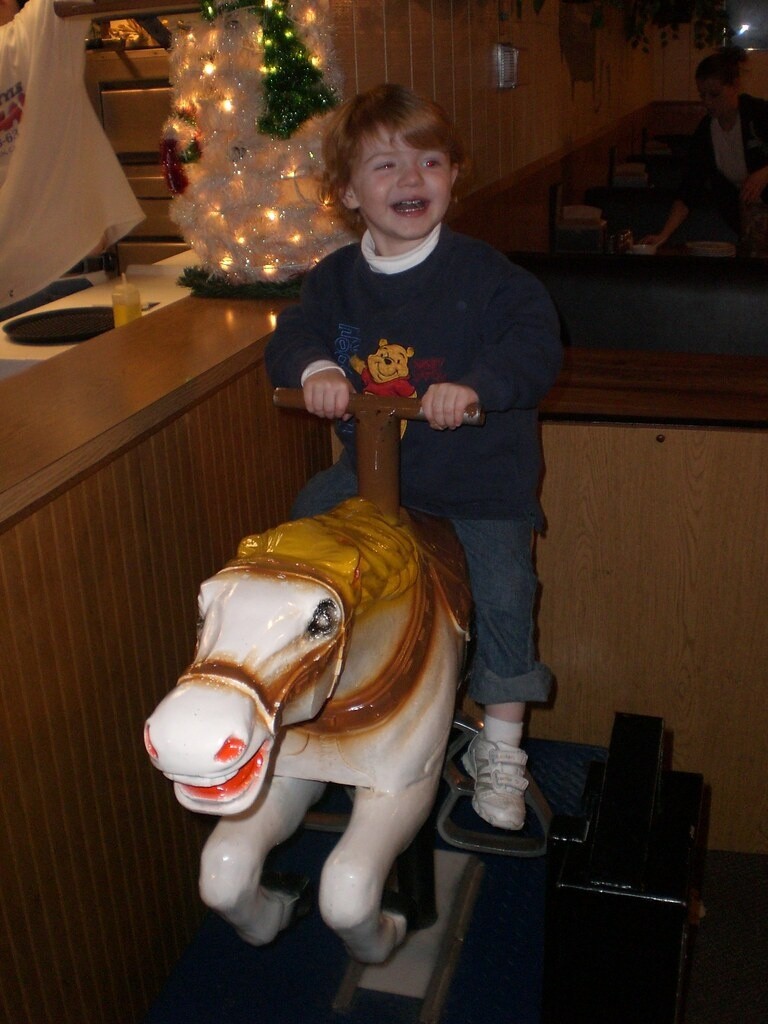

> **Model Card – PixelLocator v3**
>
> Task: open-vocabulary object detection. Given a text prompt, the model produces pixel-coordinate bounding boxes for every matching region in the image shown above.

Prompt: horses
[144,494,479,968]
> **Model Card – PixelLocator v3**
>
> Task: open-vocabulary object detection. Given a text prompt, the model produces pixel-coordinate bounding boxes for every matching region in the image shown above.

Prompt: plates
[685,240,736,258]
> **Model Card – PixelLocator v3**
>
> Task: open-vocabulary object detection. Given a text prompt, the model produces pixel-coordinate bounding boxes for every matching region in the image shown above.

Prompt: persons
[264,79,563,836]
[641,43,768,281]
[0,0,146,320]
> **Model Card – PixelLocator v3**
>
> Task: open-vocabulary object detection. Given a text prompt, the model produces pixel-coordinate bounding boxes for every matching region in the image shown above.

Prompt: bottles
[607,227,634,254]
[112,272,141,331]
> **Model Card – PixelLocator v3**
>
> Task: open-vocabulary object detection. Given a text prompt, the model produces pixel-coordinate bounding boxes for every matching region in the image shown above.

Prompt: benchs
[626,153,677,173]
[651,134,690,150]
[504,250,768,355]
[584,186,739,242]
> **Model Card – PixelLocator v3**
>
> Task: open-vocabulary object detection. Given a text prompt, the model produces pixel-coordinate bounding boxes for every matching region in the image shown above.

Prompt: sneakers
[462,730,529,831]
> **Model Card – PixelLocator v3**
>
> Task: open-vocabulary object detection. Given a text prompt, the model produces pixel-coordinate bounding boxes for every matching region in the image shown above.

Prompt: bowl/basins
[631,243,658,255]
